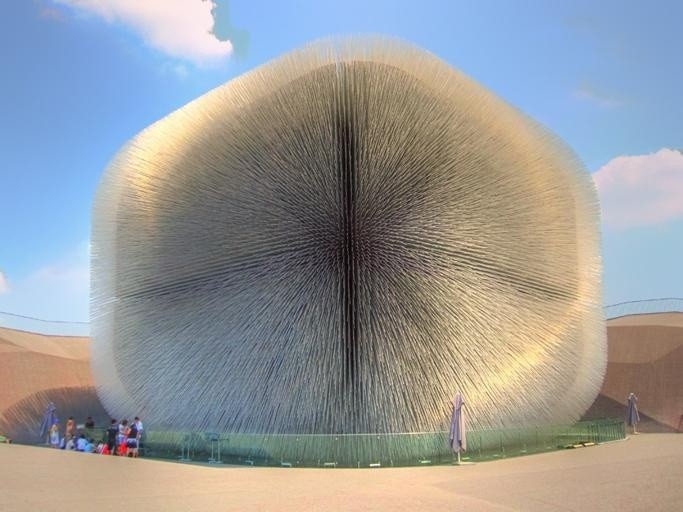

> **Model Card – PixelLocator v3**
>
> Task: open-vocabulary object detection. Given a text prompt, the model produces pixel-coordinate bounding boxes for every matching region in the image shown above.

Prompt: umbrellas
[39,403,57,443]
[448,393,466,463]
[625,393,640,432]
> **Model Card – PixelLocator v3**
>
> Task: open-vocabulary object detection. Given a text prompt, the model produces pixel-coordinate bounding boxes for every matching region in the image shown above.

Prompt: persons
[47,416,144,457]
